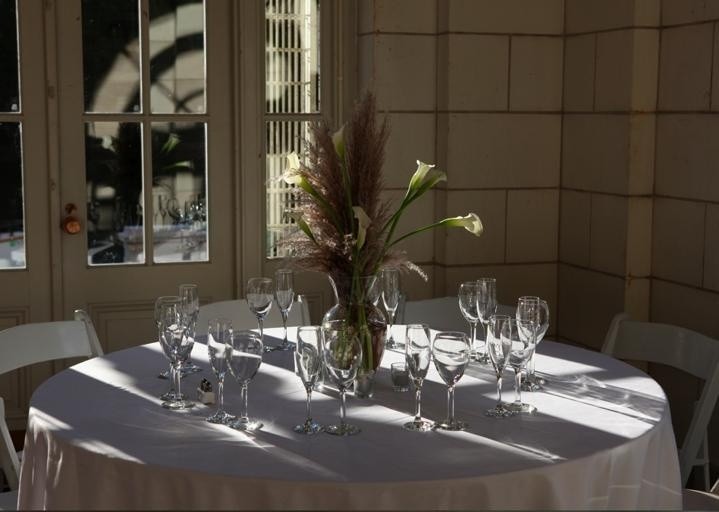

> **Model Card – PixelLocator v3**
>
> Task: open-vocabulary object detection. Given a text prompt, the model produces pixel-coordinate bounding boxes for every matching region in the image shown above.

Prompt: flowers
[270,89,483,373]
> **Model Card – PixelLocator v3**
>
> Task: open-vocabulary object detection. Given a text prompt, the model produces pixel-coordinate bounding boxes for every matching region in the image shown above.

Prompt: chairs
[602,312,719,489]
[197,295,310,330]
[404,295,517,333]
[0,308,104,489]
[682,478,719,511]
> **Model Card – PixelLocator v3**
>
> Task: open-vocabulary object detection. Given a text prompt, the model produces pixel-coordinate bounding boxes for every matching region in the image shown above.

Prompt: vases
[321,274,387,389]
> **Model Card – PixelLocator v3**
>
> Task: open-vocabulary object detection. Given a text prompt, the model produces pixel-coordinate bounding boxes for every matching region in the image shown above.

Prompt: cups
[391,363,409,391]
[355,368,373,398]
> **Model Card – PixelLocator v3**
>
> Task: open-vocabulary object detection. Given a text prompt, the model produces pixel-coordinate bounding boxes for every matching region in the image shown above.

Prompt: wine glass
[153,282,200,411]
[456,276,550,419]
[435,332,470,430]
[324,338,361,436]
[207,320,235,424]
[246,277,277,351]
[224,330,264,430]
[293,326,323,436]
[276,270,295,351]
[152,194,206,229]
[384,268,400,350]
[406,323,432,432]
